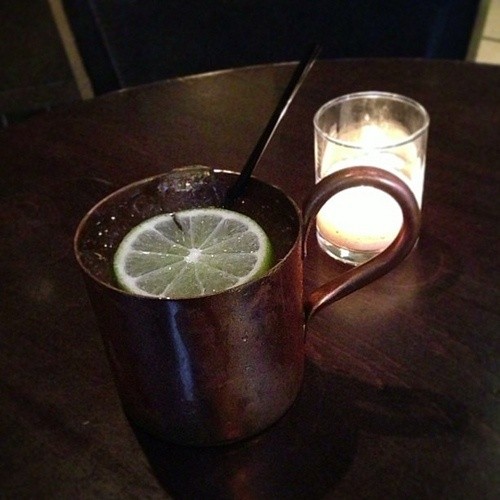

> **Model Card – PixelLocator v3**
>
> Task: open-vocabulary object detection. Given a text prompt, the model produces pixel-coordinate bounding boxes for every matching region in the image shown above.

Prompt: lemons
[115,207,273,297]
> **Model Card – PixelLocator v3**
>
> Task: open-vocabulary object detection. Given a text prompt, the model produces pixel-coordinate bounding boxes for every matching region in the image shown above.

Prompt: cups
[312,90,431,270]
[74,166,421,449]
